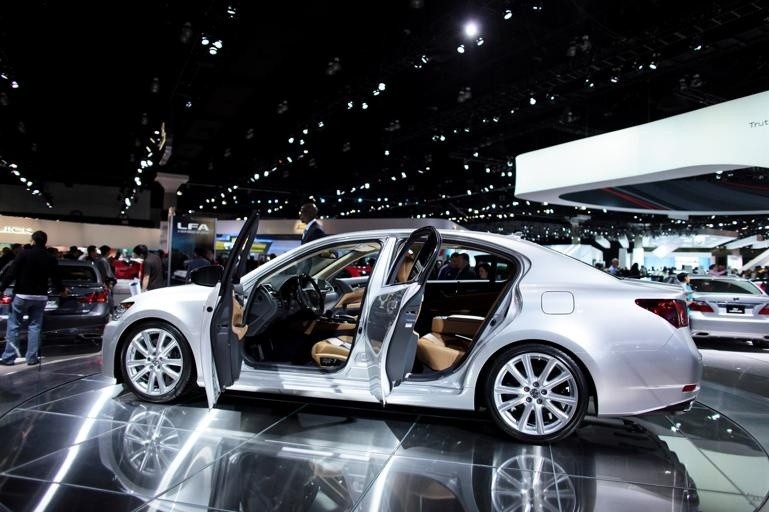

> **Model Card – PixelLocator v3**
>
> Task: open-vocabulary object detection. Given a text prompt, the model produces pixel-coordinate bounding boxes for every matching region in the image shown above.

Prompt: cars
[100,389,701,512]
[0,259,117,351]
[101,226,703,445]
[663,274,769,349]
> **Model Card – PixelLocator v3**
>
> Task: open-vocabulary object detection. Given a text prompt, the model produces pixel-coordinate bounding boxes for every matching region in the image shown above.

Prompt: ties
[303,229,308,239]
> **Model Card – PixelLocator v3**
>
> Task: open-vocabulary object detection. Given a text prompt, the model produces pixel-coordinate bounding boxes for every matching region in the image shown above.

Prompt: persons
[428,252,505,279]
[595,258,769,293]
[0,230,166,365]
[299,203,326,245]
[173,247,276,283]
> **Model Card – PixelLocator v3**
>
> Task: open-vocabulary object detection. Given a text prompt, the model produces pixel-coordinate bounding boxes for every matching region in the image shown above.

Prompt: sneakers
[27,358,40,365]
[0,358,14,366]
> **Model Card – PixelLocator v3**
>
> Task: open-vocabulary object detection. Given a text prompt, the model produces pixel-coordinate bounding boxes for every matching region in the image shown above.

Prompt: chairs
[417,311,487,372]
[308,254,414,372]
